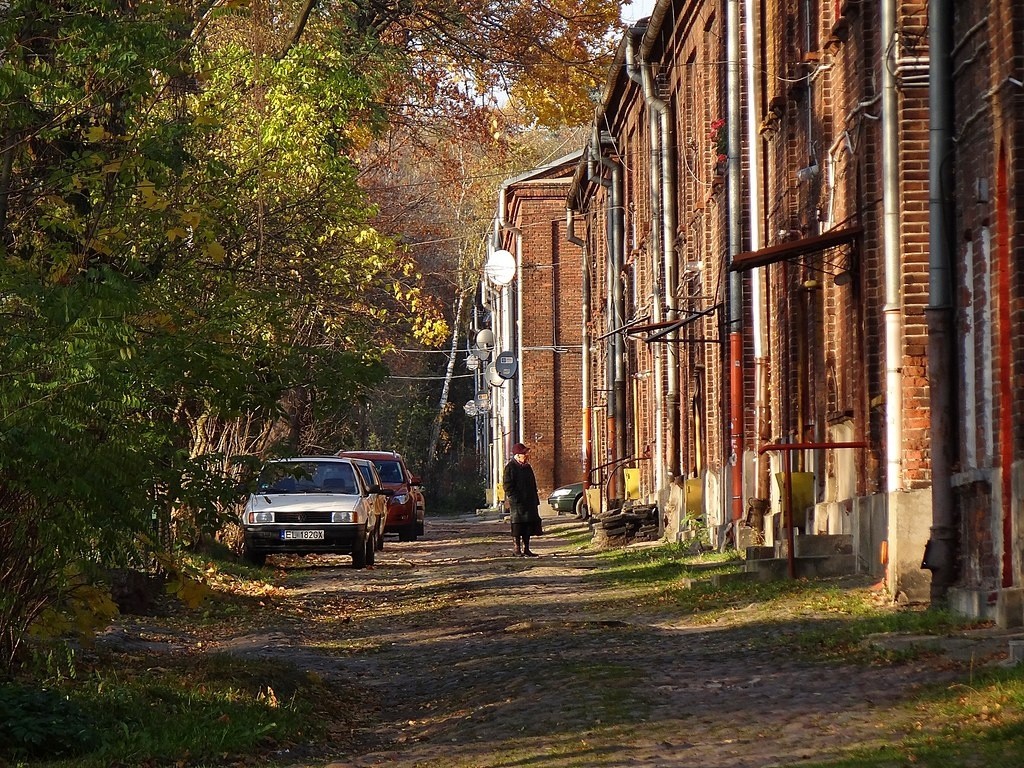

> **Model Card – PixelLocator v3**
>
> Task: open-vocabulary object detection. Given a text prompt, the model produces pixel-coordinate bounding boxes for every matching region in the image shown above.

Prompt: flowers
[707,117,729,162]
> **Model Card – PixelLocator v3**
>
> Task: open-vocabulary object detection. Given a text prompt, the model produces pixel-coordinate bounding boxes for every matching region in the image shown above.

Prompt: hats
[512,443,530,455]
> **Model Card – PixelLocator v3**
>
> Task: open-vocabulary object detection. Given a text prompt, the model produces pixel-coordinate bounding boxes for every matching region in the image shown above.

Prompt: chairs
[324,479,346,491]
[278,478,296,491]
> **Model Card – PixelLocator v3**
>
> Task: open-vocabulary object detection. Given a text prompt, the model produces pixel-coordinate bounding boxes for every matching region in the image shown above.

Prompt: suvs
[316,448,423,542]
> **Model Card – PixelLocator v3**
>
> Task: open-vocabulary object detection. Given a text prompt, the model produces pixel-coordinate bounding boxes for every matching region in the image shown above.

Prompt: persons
[503,443,541,557]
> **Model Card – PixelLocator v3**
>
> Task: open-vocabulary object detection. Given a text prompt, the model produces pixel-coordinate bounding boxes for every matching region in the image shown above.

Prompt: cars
[393,468,427,536]
[241,456,380,570]
[547,481,585,517]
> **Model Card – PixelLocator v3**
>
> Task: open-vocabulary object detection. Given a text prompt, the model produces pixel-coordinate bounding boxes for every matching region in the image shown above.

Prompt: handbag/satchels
[530,516,543,536]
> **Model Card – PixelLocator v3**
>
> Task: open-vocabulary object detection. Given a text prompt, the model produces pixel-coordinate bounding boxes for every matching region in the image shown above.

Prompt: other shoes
[512,549,522,557]
[523,549,539,557]
